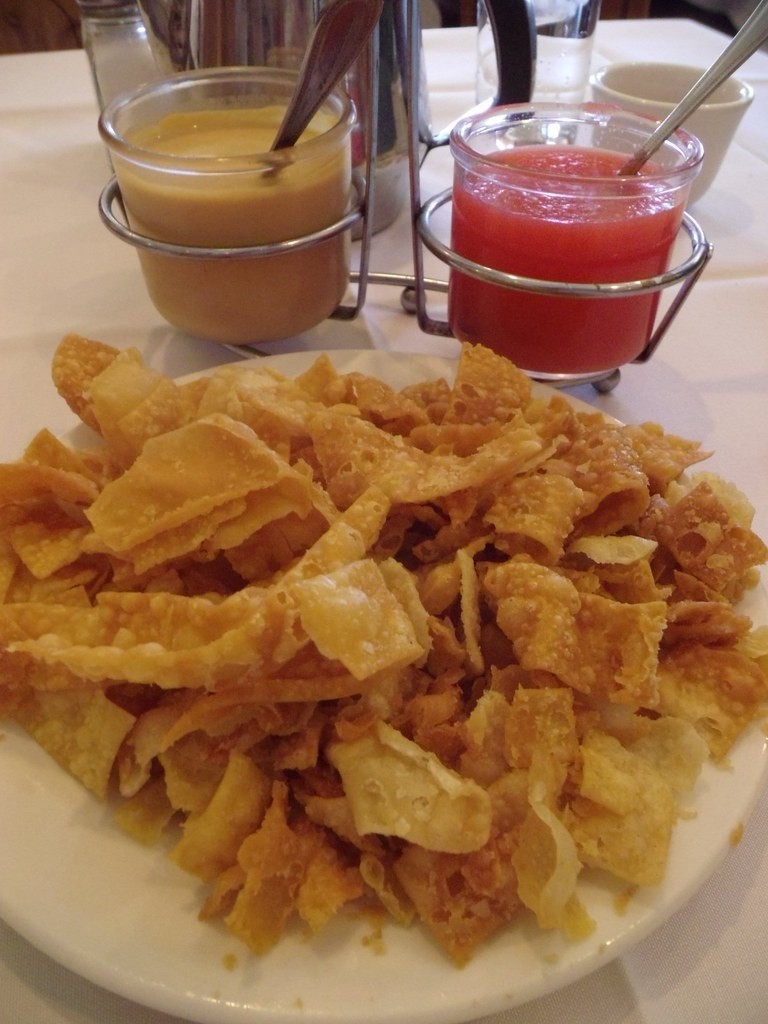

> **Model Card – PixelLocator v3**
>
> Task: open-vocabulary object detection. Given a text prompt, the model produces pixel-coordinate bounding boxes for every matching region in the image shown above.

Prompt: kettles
[136,0,538,242]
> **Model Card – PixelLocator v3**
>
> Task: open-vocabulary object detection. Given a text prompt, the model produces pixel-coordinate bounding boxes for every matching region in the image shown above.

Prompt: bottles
[79,0,172,113]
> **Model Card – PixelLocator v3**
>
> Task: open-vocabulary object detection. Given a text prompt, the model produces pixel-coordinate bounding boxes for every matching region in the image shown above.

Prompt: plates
[0,350,767,1024]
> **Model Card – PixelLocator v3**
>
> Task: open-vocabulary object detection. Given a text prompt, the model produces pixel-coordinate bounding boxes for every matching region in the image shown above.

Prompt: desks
[0,17,768,1024]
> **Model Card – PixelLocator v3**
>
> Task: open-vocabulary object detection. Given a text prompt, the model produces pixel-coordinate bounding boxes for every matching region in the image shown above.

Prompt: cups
[589,61,755,213]
[97,67,359,347]
[446,100,704,373]
[476,1,601,152]
